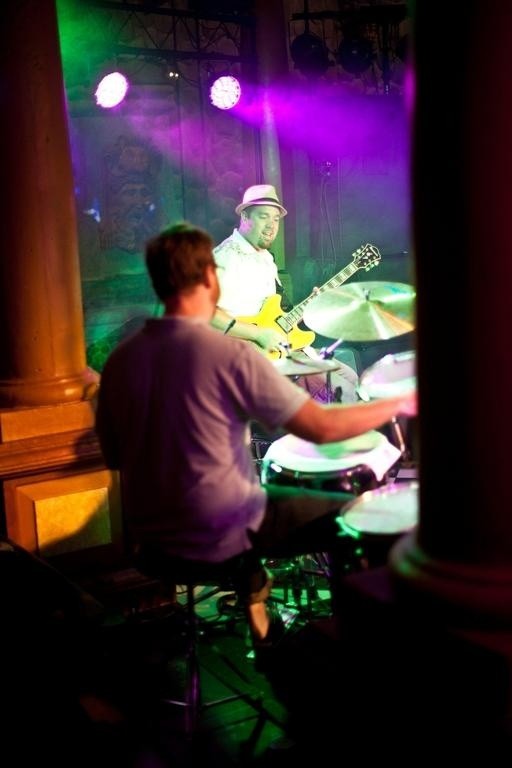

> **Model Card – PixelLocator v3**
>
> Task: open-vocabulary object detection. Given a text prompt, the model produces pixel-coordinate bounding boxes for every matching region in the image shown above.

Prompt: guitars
[234,243,382,361]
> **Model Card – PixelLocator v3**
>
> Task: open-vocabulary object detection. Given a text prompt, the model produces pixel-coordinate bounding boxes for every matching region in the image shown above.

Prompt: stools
[97,538,268,767]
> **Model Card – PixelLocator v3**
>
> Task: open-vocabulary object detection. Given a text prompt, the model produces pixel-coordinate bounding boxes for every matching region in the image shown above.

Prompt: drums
[328,481,419,616]
[262,428,402,556]
[355,348,417,469]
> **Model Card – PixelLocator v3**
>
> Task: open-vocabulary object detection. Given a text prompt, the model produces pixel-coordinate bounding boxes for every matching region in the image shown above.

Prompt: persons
[204,184,360,403]
[88,223,419,671]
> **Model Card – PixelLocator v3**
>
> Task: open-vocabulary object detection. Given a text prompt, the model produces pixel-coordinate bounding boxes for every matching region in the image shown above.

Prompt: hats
[235,185,287,218]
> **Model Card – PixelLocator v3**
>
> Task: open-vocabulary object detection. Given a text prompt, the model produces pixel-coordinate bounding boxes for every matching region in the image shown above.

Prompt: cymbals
[303,281,416,341]
[269,352,342,375]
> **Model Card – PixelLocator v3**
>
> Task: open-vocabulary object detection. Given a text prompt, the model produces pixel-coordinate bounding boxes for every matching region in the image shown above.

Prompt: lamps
[94,67,129,110]
[203,72,241,110]
